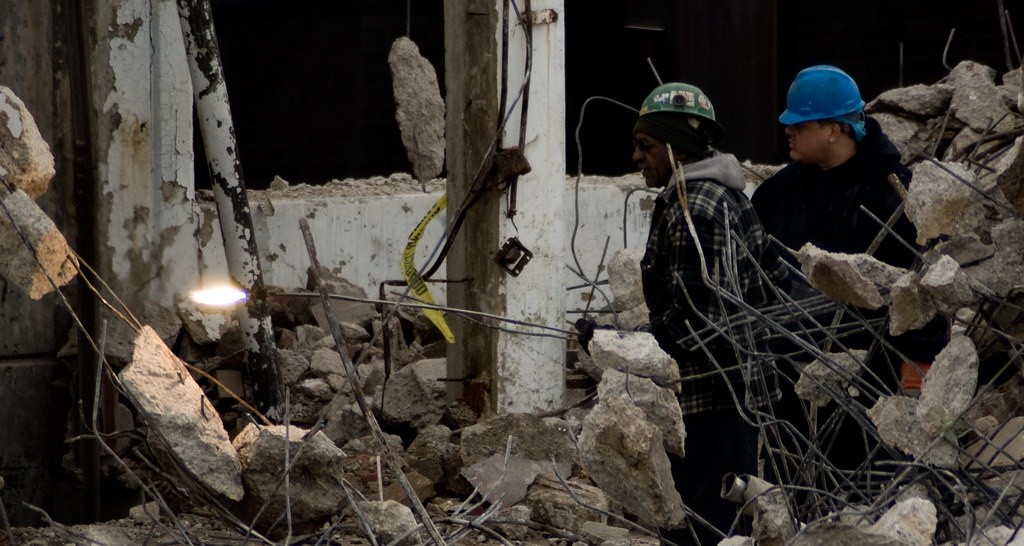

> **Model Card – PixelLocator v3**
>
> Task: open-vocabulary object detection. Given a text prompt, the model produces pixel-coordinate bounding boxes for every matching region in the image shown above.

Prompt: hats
[633,114,709,156]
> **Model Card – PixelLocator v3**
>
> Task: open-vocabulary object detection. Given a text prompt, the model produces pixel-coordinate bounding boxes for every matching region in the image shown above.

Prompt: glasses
[633,138,663,153]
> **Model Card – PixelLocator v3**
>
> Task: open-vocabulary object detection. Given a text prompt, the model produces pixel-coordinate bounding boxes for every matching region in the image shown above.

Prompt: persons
[632,83,792,545]
[750,65,950,525]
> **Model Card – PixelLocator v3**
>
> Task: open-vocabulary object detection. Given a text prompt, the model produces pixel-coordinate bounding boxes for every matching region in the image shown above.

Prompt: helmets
[639,82,723,139]
[779,64,865,126]
